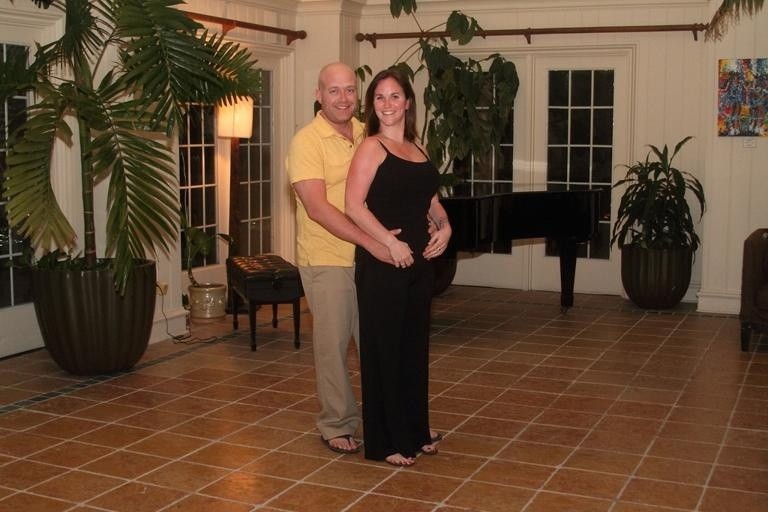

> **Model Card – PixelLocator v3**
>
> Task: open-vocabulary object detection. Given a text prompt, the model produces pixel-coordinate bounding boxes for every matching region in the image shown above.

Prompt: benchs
[225,253,305,351]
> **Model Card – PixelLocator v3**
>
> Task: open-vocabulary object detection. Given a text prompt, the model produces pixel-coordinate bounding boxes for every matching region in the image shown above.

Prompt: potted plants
[1,1,264,376]
[612,136,709,311]
[177,212,235,325]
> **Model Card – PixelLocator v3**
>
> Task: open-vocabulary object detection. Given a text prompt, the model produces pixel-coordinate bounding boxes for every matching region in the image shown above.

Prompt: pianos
[439,190,600,305]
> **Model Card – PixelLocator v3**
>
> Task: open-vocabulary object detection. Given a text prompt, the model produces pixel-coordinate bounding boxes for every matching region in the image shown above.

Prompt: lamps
[215,93,255,141]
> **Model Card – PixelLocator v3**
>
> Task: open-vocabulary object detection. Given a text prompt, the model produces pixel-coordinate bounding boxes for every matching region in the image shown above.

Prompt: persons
[344,69,452,466]
[288,63,439,455]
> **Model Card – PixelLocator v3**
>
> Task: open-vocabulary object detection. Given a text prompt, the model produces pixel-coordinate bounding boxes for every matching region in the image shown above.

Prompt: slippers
[430,432,442,440]
[320,434,359,453]
